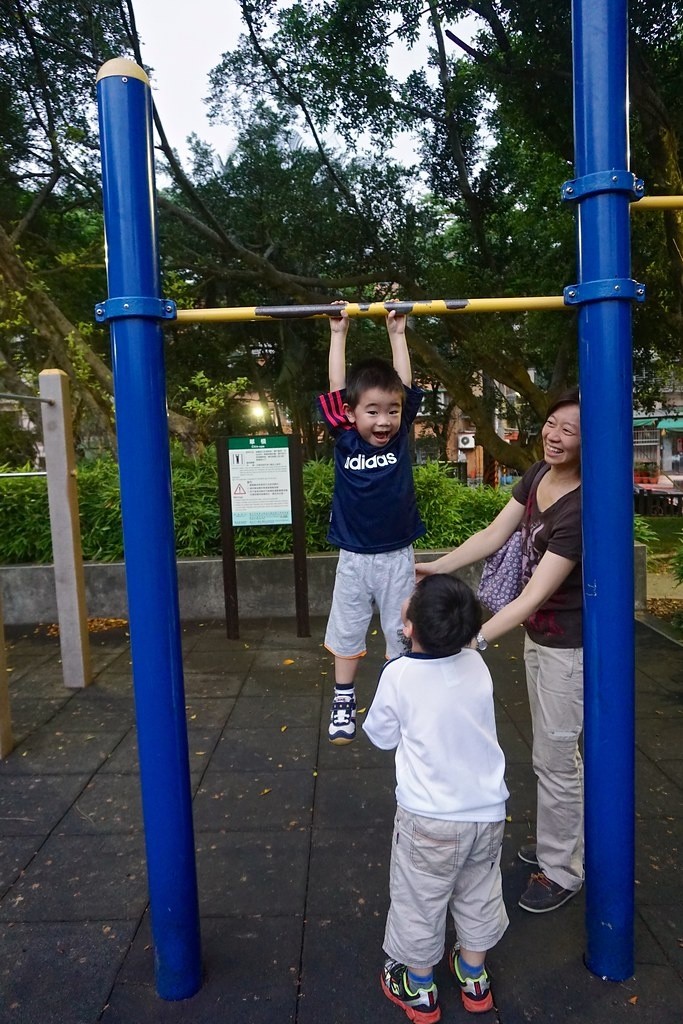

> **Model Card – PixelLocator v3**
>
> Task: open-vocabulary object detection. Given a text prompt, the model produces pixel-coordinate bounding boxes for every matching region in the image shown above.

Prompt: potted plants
[633,462,658,484]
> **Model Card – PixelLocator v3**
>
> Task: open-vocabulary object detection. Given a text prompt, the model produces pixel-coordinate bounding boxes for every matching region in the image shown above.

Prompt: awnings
[633,418,683,432]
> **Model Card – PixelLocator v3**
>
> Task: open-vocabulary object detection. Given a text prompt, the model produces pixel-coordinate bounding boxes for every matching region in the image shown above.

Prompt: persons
[362,571,509,1024]
[416,387,586,912]
[321,298,419,746]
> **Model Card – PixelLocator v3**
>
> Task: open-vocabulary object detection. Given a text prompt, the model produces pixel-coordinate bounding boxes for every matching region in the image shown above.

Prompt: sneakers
[517,870,582,914]
[378,955,442,1024]
[445,940,494,1014]
[327,687,358,746]
[518,843,539,865]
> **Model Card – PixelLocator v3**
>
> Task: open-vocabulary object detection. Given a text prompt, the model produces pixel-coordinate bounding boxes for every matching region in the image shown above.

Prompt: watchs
[475,632,488,651]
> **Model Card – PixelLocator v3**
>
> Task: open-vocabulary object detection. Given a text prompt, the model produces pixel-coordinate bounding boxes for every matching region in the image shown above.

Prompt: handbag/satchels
[475,532,523,617]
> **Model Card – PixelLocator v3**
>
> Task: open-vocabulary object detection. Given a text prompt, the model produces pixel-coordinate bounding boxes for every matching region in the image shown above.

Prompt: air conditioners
[456,434,476,449]
